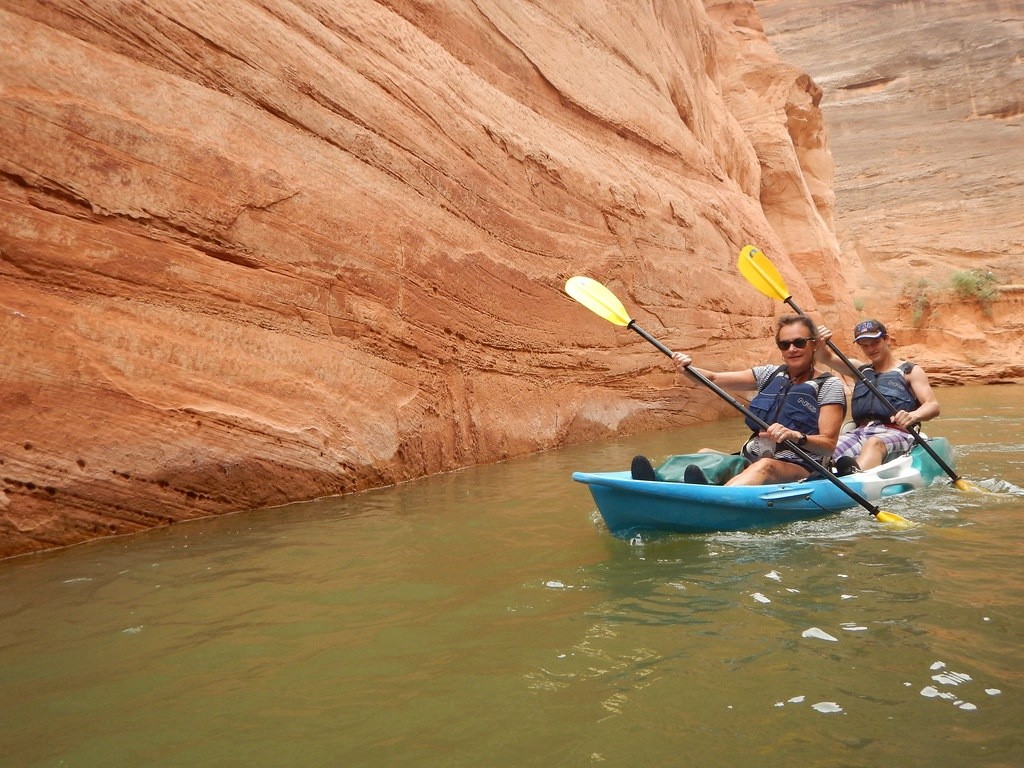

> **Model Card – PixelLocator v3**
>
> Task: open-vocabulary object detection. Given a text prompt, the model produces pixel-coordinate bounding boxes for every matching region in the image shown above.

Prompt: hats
[853,319,886,343]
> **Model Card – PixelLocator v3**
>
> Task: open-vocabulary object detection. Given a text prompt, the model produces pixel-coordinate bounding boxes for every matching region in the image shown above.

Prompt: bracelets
[796,431,807,445]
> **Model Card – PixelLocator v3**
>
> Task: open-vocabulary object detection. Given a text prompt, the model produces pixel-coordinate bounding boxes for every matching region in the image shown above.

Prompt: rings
[681,356,685,359]
[776,432,780,436]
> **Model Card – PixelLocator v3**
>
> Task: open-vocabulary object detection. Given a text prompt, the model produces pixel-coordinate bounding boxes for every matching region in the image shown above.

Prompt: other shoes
[630,455,655,480]
[836,456,861,474]
[684,464,709,484]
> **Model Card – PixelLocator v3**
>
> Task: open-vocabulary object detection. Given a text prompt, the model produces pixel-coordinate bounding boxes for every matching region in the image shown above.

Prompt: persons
[632,315,847,486]
[814,319,940,477]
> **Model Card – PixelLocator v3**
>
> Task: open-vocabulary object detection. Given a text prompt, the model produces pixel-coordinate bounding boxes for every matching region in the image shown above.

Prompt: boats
[572,437,953,537]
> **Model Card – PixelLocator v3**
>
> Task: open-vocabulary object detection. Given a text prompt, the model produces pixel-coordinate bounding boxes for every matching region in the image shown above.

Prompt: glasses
[777,337,815,350]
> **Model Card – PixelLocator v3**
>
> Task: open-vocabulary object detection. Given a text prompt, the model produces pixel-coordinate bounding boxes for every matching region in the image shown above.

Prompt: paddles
[562,273,912,526]
[736,244,979,492]
